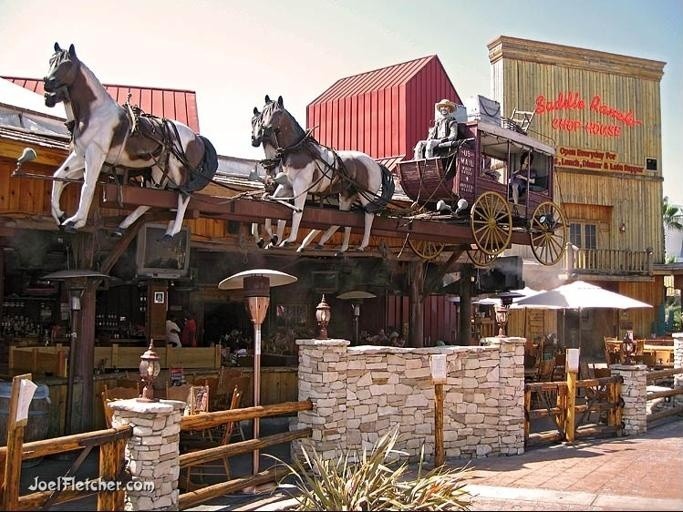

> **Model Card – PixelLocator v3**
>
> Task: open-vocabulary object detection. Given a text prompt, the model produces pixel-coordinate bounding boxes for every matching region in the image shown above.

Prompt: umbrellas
[444,278,656,395]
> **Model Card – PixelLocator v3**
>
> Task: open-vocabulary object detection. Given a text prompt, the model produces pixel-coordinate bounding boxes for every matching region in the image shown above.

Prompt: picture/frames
[153,291,164,304]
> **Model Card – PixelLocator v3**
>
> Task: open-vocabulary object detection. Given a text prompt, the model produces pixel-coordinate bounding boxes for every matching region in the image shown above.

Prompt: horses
[43,42,218,242]
[251,94,395,256]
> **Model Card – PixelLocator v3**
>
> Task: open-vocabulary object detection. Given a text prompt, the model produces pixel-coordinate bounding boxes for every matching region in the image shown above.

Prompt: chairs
[437,123,465,162]
[524,176,548,192]
[236,352,255,368]
[102,379,245,493]
[523,335,672,427]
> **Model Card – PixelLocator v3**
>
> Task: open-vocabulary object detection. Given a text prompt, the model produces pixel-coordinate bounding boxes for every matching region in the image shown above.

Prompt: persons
[176,308,198,347]
[507,150,537,204]
[412,97,459,160]
[165,309,183,347]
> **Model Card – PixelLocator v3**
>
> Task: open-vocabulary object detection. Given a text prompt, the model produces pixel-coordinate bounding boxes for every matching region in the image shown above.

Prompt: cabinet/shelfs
[0,284,150,340]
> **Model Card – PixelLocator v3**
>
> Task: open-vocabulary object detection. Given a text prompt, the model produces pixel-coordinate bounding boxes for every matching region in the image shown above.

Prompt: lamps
[315,293,333,340]
[496,304,509,337]
[619,224,626,232]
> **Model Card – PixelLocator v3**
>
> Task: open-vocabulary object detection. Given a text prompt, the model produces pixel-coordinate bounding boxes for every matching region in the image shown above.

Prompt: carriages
[9,41,567,267]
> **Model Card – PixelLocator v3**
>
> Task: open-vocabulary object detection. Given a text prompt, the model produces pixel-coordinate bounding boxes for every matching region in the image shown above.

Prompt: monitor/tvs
[135,223,191,278]
[311,271,338,294]
